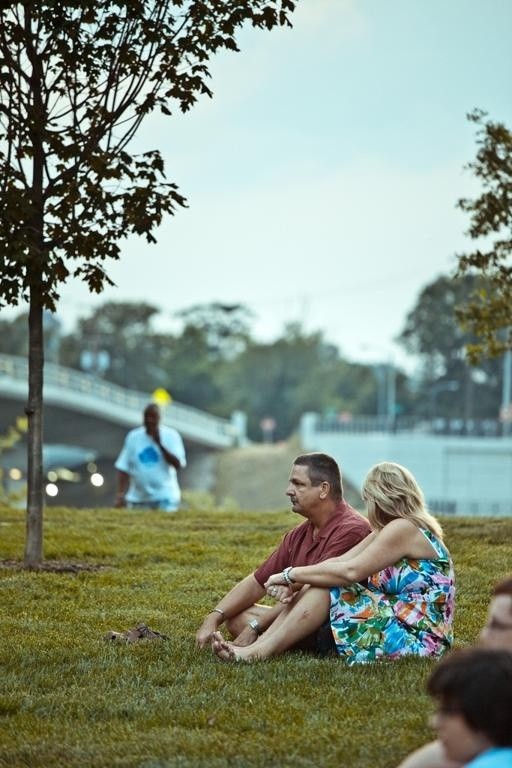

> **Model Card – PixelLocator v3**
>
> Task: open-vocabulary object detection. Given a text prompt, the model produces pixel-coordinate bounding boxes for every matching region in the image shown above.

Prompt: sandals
[105,622,169,643]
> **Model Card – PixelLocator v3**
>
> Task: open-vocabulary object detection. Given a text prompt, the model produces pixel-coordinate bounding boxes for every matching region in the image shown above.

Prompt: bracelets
[212,608,225,623]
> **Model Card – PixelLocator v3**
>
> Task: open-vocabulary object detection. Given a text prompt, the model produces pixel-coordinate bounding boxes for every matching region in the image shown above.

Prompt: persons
[259,415,277,445]
[195,452,374,650]
[113,404,187,512]
[426,649,512,768]
[326,405,352,429]
[212,461,457,667]
[394,577,512,768]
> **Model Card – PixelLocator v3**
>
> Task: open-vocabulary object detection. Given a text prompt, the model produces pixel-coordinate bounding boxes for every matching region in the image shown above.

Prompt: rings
[273,589,277,592]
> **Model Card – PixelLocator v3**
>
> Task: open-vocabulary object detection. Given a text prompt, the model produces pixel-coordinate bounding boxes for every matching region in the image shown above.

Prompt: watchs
[283,567,297,585]
[249,619,262,636]
